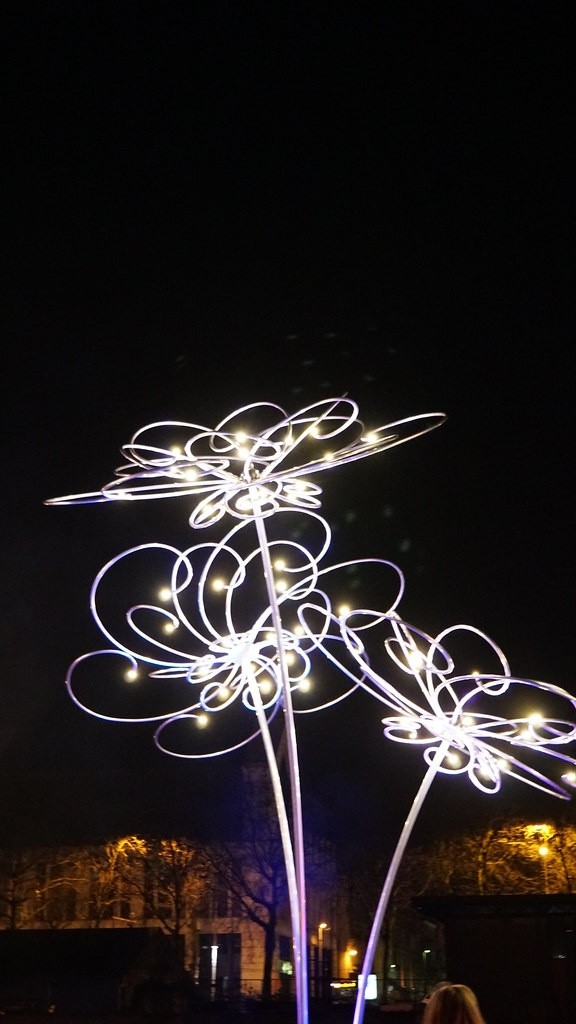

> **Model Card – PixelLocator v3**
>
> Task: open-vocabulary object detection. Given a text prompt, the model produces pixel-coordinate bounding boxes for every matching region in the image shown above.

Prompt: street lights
[532,824,550,894]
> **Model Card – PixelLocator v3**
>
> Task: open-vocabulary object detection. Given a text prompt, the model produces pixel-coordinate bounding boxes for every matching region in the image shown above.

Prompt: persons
[424,983,488,1024]
